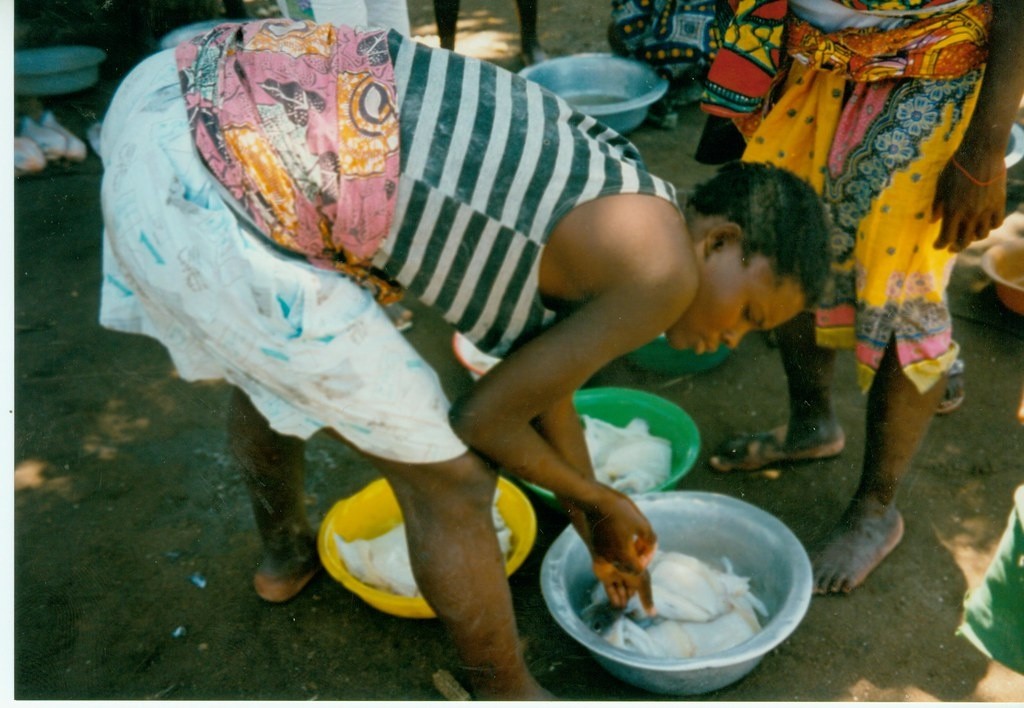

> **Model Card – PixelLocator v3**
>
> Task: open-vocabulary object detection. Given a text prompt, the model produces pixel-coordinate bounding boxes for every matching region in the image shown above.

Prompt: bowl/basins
[540,490,815,696]
[1006,122,1024,166]
[521,384,700,516]
[14,43,108,96]
[624,336,736,371]
[162,19,228,47]
[453,330,502,381]
[317,476,537,619]
[517,54,670,134]
[978,239,1024,315]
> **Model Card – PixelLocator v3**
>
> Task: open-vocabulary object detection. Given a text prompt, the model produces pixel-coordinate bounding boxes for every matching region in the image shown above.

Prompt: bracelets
[950,151,1006,186]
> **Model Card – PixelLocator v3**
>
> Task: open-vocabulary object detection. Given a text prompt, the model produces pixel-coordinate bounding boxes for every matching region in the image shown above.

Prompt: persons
[696,0,1024,594]
[608,0,734,127]
[434,0,543,67]
[100,20,833,701]
